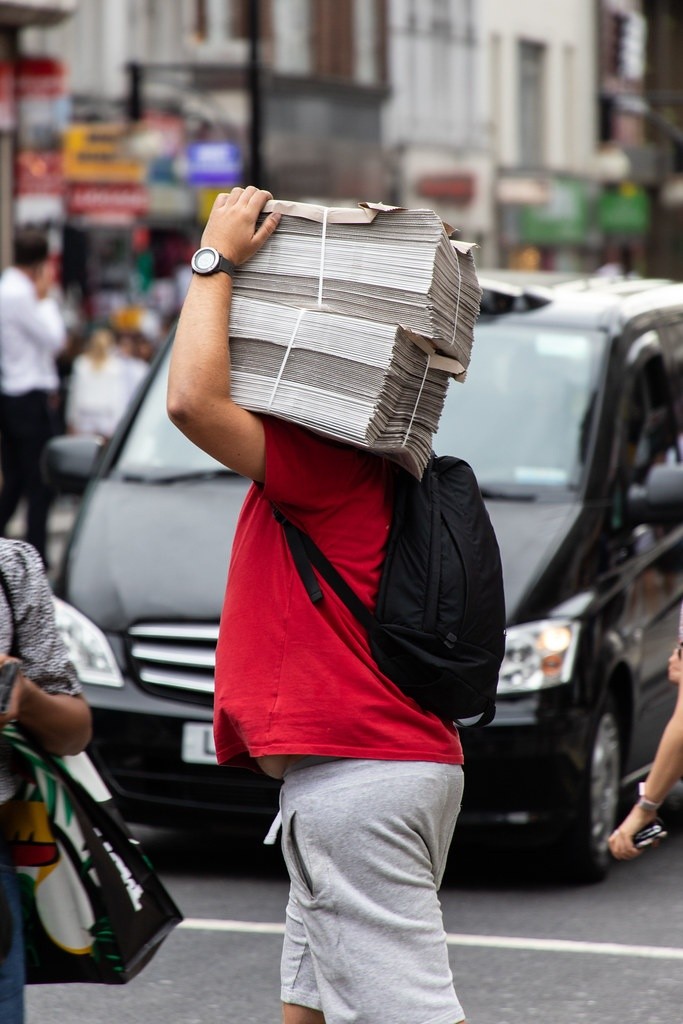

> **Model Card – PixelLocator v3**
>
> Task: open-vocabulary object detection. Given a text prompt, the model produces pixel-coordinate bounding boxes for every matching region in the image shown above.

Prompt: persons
[165,183,466,1024]
[0,233,69,573]
[64,305,193,440]
[608,599,683,861]
[0,534,93,1024]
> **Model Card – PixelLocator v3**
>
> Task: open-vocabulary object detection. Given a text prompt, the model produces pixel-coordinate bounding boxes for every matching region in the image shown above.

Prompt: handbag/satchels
[5,732,185,986]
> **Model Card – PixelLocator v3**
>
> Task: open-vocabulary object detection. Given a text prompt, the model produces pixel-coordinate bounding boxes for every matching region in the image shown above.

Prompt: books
[225,205,482,483]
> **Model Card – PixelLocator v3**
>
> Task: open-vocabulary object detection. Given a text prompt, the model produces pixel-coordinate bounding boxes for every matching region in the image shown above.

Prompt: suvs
[36,263,683,888]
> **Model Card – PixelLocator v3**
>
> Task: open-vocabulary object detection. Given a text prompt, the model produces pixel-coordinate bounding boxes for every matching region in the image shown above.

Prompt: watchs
[191,246,237,280]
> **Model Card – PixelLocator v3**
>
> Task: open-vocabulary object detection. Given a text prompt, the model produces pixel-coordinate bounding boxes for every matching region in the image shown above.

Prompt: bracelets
[638,782,664,811]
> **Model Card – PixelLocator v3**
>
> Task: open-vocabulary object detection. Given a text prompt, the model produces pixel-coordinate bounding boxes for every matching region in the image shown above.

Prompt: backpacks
[258,441,507,727]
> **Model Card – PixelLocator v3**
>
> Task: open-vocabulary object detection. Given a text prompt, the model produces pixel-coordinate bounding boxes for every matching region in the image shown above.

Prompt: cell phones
[0,662,20,713]
[634,831,668,849]
